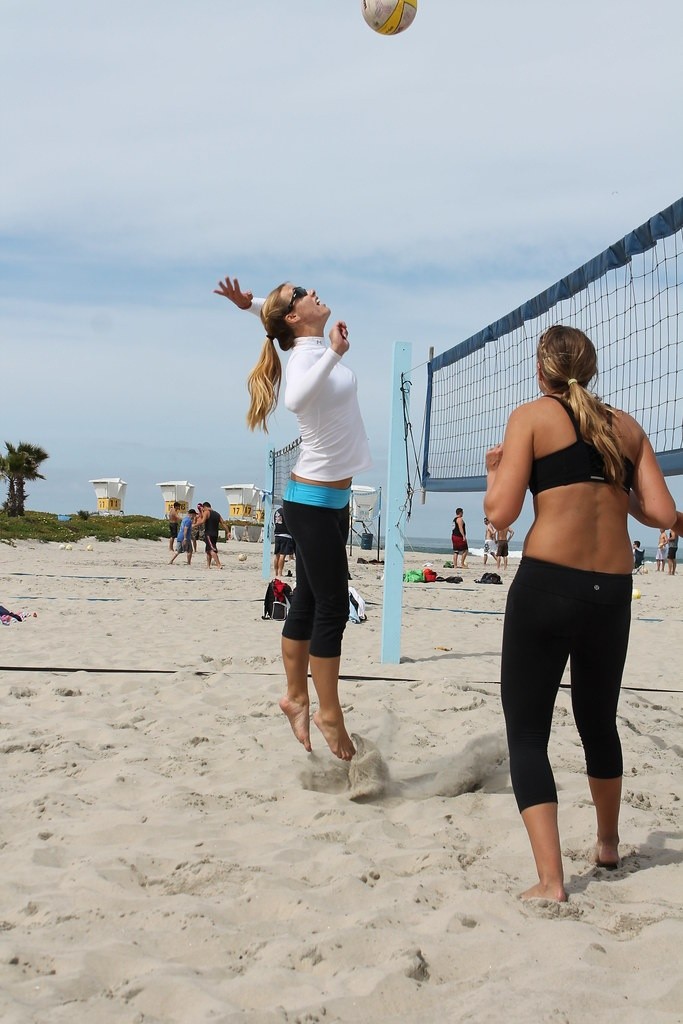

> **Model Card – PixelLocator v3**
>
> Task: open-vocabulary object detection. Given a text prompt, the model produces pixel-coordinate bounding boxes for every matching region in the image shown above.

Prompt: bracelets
[183,538,187,540]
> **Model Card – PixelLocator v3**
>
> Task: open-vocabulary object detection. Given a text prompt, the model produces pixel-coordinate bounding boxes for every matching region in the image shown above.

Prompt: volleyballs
[361,0,417,35]
[631,589,640,600]
[238,554,247,560]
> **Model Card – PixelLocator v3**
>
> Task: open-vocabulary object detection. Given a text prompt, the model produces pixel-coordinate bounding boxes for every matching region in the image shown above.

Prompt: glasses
[286,286,308,315]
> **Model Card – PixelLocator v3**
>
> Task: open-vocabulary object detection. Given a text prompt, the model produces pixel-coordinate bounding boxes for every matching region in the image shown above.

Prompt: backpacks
[262,579,292,621]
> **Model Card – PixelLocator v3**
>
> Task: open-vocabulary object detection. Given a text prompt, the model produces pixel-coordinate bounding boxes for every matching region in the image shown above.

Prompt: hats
[188,509,198,515]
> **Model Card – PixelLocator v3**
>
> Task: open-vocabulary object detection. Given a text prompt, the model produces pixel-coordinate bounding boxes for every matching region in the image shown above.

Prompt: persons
[167,509,200,566]
[482,323,678,903]
[489,525,514,570]
[655,528,668,573]
[191,503,207,554]
[671,510,683,539]
[451,508,469,569]
[191,502,232,570]
[666,528,680,576]
[273,507,293,576]
[480,517,501,565]
[631,540,643,568]
[212,275,359,761]
[168,501,184,552]
[288,544,295,561]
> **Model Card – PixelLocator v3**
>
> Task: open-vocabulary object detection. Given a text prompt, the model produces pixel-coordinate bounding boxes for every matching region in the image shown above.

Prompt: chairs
[633,549,645,575]
